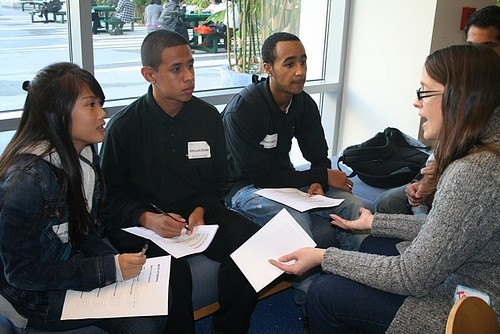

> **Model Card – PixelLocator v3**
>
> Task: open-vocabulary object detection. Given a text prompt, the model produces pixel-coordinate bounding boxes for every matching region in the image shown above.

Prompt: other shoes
[197,42,210,47]
[45,21,48,23]
[189,38,195,43]
[93,28,97,34]
[293,287,306,306]
[99,25,104,28]
[38,15,43,18]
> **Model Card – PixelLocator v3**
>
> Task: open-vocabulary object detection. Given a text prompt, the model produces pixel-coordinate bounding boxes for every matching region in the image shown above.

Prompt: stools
[20,1,40,22]
[105,20,122,35]
[131,17,142,32]
[57,12,66,23]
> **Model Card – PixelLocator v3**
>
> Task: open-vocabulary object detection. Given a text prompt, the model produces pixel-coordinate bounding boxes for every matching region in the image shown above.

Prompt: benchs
[1,155,388,334]
[195,33,234,53]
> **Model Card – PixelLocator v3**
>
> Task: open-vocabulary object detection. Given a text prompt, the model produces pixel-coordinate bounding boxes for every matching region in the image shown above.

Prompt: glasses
[417,88,443,102]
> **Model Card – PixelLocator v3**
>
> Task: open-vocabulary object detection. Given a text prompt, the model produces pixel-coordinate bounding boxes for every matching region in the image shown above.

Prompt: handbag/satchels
[337,127,431,187]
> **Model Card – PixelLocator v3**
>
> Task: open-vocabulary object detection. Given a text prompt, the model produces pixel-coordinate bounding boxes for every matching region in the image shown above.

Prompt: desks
[182,13,213,45]
[35,1,56,22]
[93,5,116,29]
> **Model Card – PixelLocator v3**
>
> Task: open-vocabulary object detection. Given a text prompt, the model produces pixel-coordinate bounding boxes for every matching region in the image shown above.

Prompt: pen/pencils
[141,242,148,255]
[347,183,353,188]
[153,205,190,230]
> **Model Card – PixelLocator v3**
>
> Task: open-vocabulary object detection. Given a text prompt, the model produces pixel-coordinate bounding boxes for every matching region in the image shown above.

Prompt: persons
[92,12,104,34]
[191,0,239,54]
[267,42,500,334]
[99,31,263,334]
[220,33,372,305]
[109,0,134,35]
[373,5,500,214]
[0,62,171,334]
[144,0,195,54]
[38,0,62,23]
